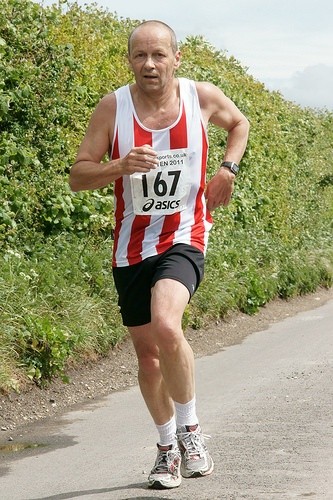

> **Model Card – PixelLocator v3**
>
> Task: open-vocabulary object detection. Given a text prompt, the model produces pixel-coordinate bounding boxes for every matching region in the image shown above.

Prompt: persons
[68,21,251,489]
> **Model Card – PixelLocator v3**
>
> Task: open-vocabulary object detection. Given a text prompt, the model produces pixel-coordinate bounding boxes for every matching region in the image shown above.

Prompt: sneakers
[175,423,216,478]
[148,444,182,490]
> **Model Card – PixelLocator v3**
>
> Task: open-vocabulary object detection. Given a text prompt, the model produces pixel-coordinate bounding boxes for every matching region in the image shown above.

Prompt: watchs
[220,161,240,175]
